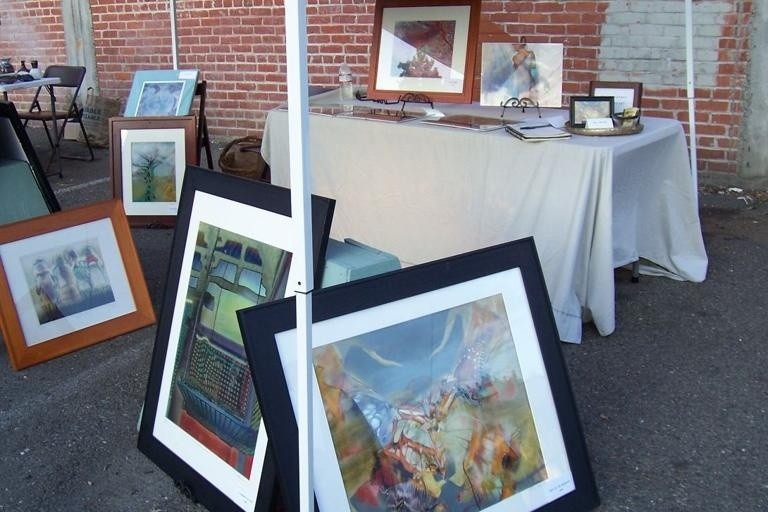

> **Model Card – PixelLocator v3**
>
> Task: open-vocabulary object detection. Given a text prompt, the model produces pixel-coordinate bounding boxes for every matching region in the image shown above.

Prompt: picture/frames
[137,164,336,511]
[123,69,199,117]
[570,96,614,127]
[236,236,599,511]
[366,0,481,105]
[590,81,642,115]
[108,116,196,229]
[0,100,62,213]
[0,198,157,371]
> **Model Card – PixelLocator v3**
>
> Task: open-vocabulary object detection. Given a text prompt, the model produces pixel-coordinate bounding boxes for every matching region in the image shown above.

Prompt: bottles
[337,62,353,101]
[0,57,41,85]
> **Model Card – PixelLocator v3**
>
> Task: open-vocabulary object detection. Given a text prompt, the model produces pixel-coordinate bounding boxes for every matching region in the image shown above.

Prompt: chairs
[196,80,213,169]
[18,65,95,173]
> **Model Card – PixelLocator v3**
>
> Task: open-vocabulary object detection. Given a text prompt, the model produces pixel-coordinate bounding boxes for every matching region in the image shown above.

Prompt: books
[278,99,343,118]
[336,105,428,124]
[420,112,528,134]
[505,121,573,145]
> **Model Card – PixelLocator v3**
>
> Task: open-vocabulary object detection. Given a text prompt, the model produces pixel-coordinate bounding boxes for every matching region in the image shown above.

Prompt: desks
[260,86,709,346]
[0,77,62,178]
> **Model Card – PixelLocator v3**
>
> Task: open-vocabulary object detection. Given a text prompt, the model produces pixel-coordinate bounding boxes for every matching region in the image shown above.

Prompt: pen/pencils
[520,124,553,129]
